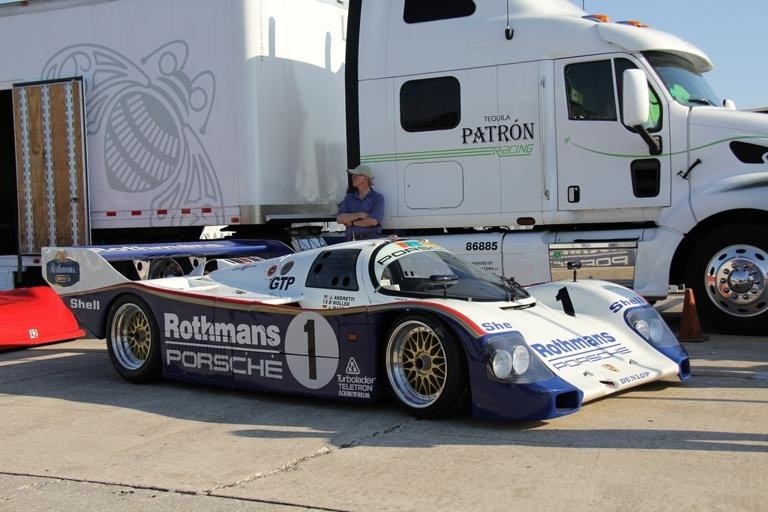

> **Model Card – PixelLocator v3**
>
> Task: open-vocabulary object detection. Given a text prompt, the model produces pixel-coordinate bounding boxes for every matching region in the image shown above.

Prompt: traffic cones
[676,288,711,344]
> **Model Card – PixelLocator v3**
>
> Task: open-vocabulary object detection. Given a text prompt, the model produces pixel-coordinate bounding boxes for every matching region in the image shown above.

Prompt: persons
[337,164,384,241]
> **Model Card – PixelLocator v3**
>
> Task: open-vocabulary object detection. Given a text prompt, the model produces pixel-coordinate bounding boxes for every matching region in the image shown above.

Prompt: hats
[345,165,372,178]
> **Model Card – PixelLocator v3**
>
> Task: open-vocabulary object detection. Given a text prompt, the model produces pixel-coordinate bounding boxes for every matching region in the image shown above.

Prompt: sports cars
[41,236,692,420]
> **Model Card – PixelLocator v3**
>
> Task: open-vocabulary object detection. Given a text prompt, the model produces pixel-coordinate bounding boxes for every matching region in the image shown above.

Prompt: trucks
[0,1,768,337]
